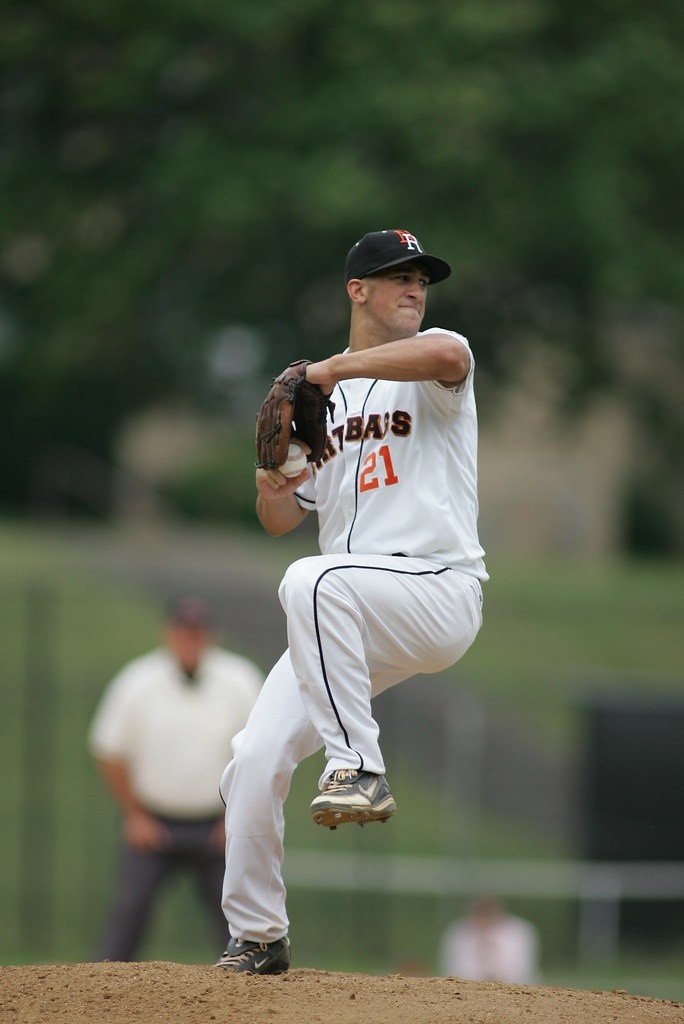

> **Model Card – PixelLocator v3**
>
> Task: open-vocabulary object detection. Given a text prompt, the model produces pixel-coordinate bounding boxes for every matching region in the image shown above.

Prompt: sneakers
[310,768,397,830]
[213,933,291,975]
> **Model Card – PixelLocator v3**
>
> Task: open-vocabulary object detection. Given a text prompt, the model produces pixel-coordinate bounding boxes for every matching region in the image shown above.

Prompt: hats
[344,230,452,285]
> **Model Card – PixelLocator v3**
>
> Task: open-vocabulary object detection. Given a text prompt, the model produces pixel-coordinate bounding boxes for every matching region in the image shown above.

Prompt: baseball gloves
[253,358,334,471]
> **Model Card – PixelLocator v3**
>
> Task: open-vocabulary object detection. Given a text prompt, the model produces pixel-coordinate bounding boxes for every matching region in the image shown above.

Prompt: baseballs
[272,442,307,479]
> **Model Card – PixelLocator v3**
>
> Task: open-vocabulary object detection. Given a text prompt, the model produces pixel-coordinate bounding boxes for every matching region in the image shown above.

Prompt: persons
[195,229,490,974]
[85,578,269,962]
